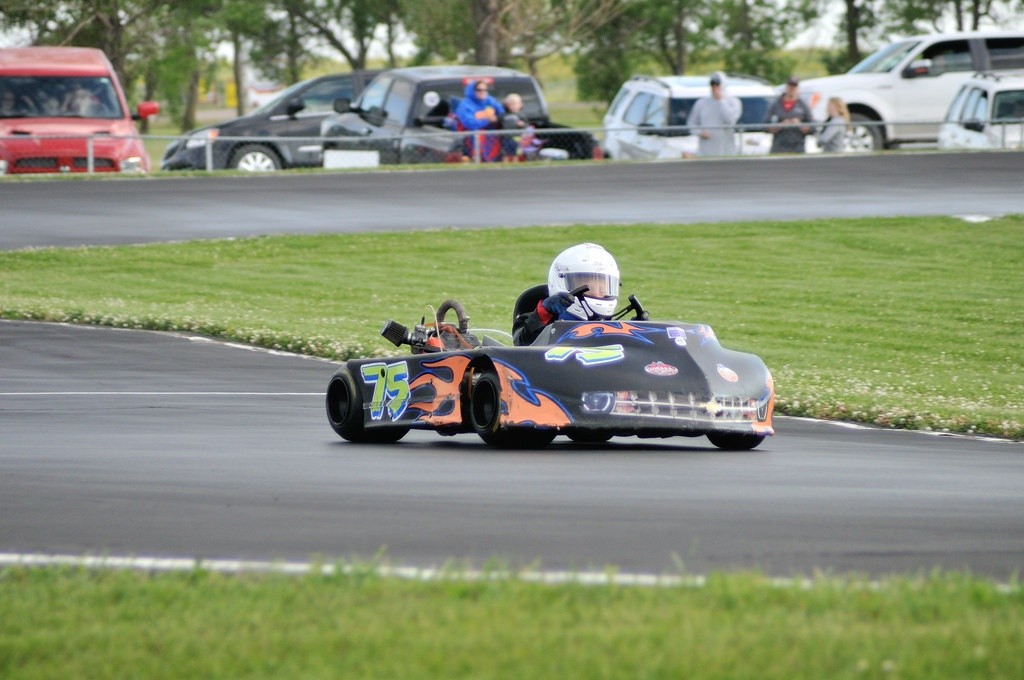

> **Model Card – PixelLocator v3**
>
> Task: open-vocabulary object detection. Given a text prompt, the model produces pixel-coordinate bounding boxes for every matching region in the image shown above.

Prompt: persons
[764,77,817,154]
[686,73,744,157]
[817,96,853,153]
[513,242,626,345]
[452,81,530,162]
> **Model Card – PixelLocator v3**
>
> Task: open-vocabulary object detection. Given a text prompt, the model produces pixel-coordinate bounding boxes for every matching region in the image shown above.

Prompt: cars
[0,47,162,174]
[159,67,393,170]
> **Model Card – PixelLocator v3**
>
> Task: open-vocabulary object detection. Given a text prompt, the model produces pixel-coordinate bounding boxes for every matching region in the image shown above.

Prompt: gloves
[631,316,648,321]
[542,292,574,314]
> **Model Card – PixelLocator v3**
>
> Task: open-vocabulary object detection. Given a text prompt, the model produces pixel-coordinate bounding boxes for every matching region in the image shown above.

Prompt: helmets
[548,243,620,321]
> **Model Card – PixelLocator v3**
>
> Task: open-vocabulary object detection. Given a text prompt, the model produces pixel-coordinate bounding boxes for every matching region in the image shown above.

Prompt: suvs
[601,74,824,161]
[775,32,1024,153]
[935,71,1024,148]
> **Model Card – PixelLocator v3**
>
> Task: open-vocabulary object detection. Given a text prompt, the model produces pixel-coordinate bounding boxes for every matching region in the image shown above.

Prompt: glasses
[711,81,720,85]
[475,88,489,91]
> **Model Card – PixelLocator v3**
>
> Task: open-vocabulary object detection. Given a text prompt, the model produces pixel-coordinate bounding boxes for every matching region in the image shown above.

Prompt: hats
[788,76,798,86]
[710,71,725,84]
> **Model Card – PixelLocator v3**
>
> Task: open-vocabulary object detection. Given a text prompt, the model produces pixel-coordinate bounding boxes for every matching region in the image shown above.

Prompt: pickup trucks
[320,64,612,169]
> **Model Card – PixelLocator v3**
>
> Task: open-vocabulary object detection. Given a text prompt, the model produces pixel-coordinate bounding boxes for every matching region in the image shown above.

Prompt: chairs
[513,285,550,323]
